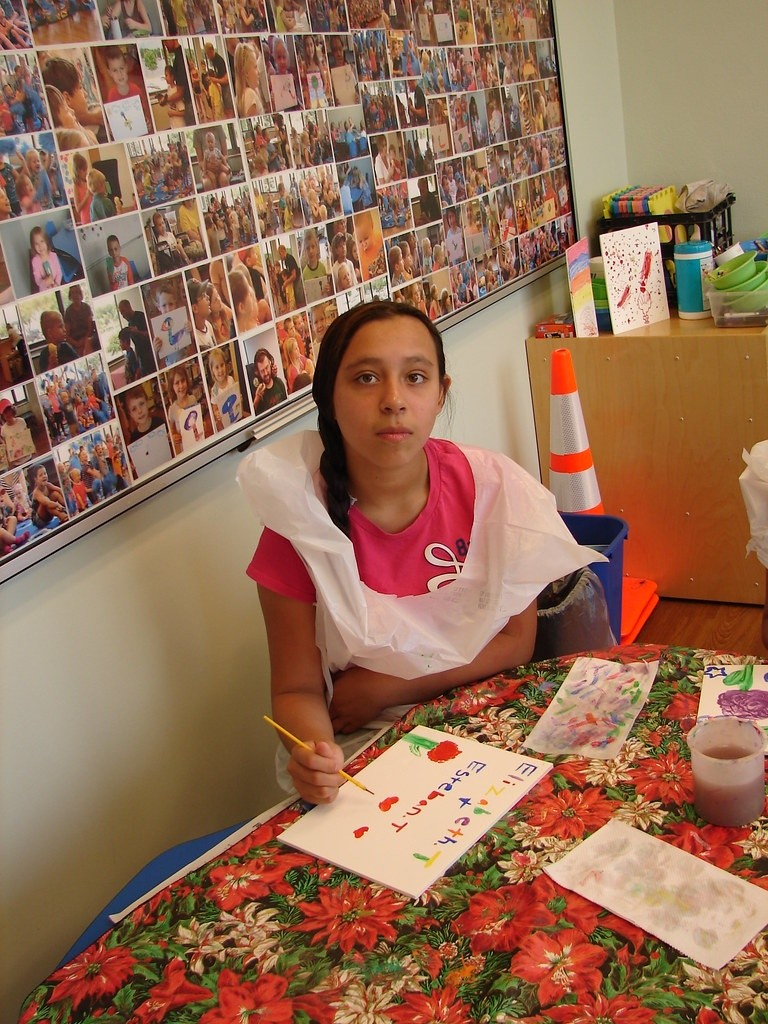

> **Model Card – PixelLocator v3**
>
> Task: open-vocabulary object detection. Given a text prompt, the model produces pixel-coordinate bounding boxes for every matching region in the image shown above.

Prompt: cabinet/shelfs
[526,308,768,607]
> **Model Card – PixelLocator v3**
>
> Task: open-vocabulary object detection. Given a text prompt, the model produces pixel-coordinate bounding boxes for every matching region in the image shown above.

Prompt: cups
[713,242,745,267]
[687,715,766,826]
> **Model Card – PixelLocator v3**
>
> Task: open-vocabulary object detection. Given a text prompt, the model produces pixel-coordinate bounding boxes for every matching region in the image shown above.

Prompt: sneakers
[2,546,14,555]
[16,531,29,546]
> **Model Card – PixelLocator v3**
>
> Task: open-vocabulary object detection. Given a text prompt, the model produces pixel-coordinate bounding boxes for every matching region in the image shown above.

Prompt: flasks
[674,240,714,320]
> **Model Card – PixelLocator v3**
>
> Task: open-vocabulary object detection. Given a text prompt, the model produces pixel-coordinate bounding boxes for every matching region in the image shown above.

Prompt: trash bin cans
[558,511,629,645]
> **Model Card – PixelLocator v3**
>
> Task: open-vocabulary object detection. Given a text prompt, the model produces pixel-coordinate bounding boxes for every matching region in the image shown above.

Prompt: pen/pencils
[754,240,765,251]
[712,244,727,258]
[703,269,724,281]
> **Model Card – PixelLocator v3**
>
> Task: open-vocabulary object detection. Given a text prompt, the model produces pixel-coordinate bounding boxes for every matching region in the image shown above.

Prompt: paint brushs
[263,715,375,795]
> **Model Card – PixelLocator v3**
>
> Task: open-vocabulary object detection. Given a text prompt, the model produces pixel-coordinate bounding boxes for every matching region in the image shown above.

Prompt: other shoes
[59,514,69,524]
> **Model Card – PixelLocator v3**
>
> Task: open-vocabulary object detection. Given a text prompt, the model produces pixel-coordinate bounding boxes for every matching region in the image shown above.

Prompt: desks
[17,646,768,1024]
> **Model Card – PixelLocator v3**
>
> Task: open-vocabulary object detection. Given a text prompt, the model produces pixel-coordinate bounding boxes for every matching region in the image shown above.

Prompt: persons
[0,0,573,555]
[236,301,610,805]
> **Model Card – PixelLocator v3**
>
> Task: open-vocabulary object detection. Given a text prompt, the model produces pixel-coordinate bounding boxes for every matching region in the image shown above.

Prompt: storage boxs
[535,313,574,339]
[595,193,737,308]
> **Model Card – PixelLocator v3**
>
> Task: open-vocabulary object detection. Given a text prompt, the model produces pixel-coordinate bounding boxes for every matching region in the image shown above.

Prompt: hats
[187,278,209,302]
[0,487,6,495]
[0,399,13,414]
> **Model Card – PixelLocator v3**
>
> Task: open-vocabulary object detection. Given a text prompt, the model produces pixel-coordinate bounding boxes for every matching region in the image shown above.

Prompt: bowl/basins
[721,278,768,313]
[715,260,768,298]
[704,250,759,288]
[589,256,612,331]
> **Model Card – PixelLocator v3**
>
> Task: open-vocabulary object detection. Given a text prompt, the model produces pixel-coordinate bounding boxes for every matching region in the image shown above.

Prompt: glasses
[197,292,207,302]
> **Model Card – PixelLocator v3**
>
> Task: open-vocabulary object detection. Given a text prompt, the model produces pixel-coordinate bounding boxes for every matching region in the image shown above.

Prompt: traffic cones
[545,348,660,654]
